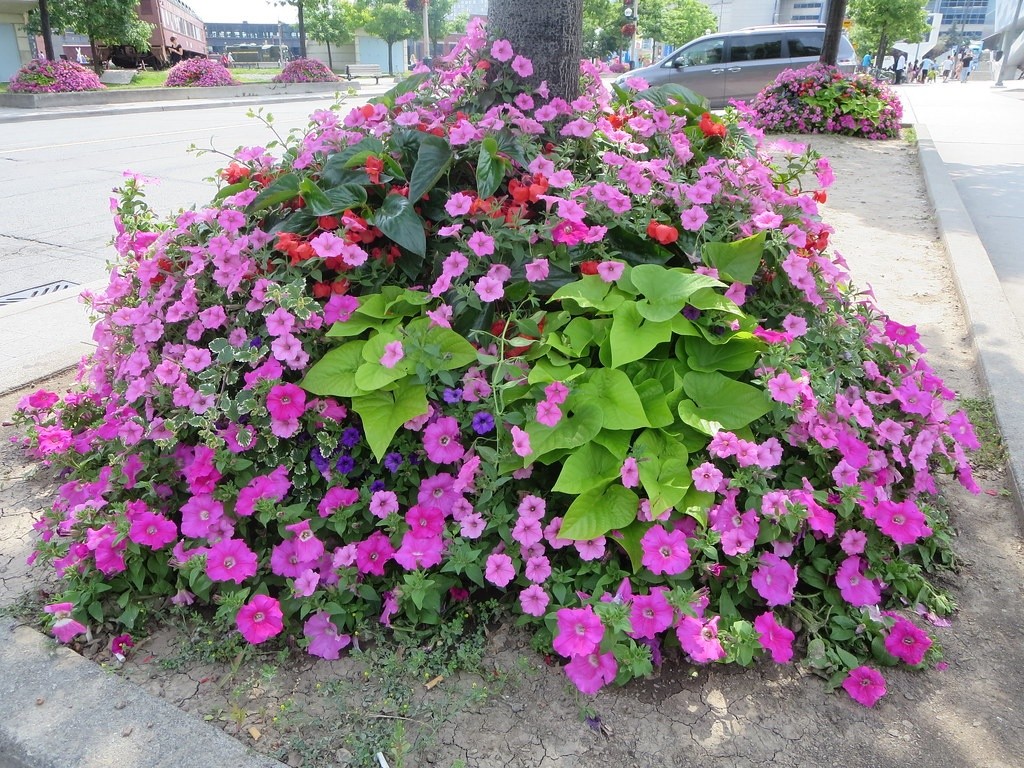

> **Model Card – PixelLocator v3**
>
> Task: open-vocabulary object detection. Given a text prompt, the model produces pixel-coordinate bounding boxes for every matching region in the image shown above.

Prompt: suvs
[611,23,859,110]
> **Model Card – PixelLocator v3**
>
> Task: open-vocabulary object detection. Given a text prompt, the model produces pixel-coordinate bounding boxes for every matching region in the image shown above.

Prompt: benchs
[343,65,383,84]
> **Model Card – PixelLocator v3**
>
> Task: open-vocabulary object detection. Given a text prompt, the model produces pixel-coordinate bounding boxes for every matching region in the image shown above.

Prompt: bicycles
[869,69,897,84]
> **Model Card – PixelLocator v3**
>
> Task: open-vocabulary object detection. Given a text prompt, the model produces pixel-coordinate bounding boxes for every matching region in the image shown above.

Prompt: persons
[862,51,871,72]
[168,36,183,66]
[906,49,952,83]
[706,45,719,64]
[410,54,416,68]
[221,52,235,67]
[896,52,905,84]
[960,45,980,82]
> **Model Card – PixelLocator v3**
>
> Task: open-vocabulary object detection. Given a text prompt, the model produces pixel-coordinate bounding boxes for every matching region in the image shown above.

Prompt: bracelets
[175,50,176,53]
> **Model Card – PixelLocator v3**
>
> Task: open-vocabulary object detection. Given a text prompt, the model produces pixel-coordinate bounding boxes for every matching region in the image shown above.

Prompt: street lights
[625,7,637,70]
